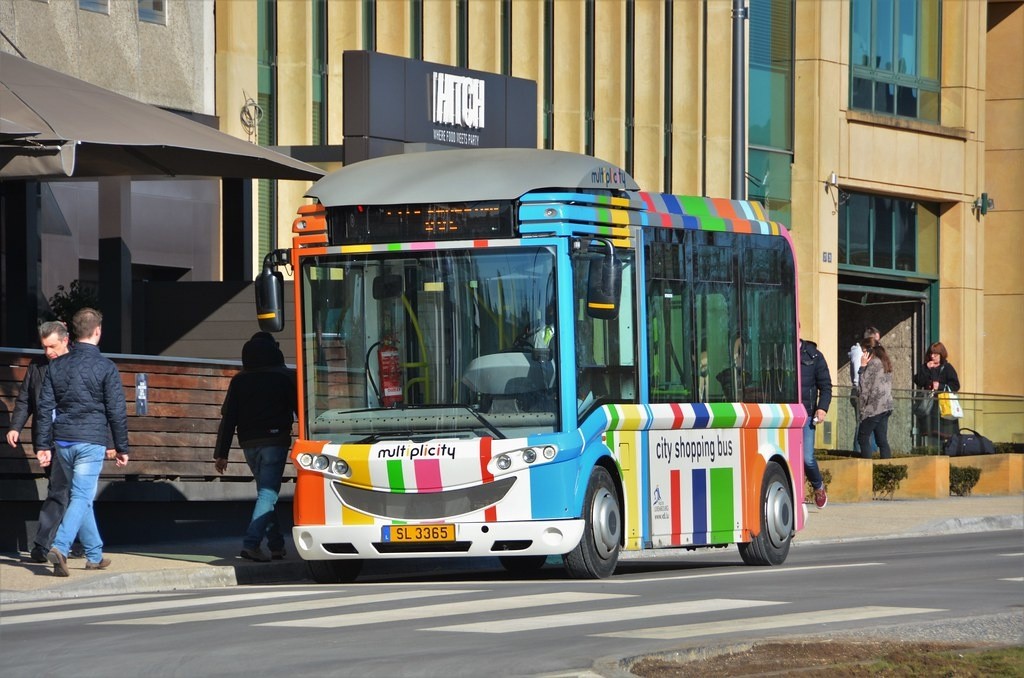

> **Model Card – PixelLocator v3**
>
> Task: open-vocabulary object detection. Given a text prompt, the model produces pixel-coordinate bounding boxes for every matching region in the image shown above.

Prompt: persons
[799,322,832,507]
[214,332,298,562]
[30,308,130,577]
[6,321,84,563]
[856,337,895,458]
[913,342,961,446]
[848,326,881,453]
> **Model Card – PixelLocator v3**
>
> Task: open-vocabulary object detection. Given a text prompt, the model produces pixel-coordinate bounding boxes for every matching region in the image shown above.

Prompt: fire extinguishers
[366,332,403,407]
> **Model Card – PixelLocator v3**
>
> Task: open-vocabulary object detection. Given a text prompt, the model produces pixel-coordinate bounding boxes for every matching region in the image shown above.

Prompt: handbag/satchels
[944,428,994,455]
[938,384,963,420]
[914,389,935,418]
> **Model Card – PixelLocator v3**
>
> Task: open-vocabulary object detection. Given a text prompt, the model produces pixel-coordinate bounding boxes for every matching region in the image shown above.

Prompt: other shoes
[69,546,85,559]
[31,547,48,563]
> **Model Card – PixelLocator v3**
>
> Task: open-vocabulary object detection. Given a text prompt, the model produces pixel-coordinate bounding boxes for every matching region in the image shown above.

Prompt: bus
[254,147,806,584]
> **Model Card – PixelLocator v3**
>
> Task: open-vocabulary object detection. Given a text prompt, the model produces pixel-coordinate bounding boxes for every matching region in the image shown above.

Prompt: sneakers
[271,548,287,559]
[240,548,266,561]
[48,547,70,577]
[814,488,828,509]
[86,558,112,570]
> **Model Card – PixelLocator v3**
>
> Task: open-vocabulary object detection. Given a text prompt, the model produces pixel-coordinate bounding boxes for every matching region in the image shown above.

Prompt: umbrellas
[0,49,328,183]
